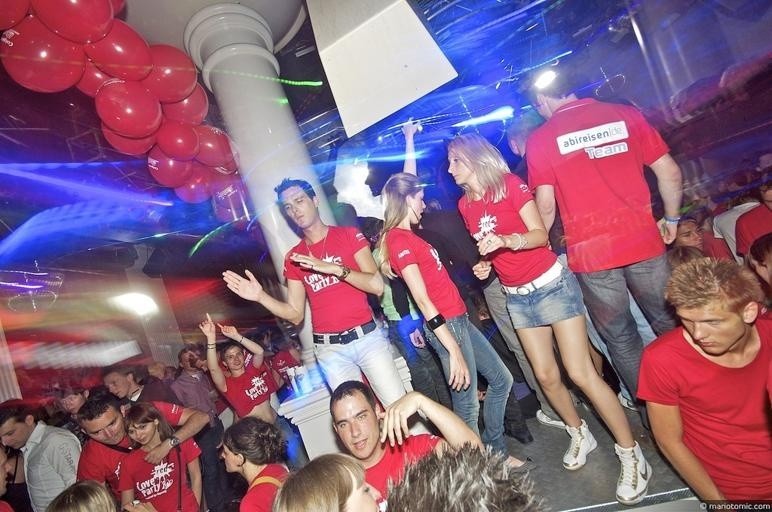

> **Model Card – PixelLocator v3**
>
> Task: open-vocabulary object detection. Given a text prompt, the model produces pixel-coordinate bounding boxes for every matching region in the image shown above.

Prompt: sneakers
[560,418,653,506]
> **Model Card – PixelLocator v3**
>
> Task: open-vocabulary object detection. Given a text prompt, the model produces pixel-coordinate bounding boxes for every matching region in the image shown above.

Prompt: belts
[501,258,563,296]
[314,321,376,344]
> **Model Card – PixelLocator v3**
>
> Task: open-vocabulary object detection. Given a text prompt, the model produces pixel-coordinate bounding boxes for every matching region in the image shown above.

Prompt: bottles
[294,365,313,394]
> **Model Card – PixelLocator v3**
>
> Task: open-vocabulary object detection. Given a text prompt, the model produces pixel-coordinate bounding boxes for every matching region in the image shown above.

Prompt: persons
[0,66,772,512]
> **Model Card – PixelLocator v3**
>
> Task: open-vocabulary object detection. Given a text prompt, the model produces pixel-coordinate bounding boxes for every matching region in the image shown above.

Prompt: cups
[287,367,299,393]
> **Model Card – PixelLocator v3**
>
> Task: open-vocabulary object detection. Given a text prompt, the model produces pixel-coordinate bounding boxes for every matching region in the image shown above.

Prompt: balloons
[0,0,256,234]
